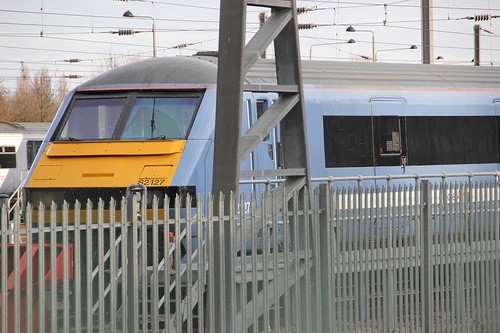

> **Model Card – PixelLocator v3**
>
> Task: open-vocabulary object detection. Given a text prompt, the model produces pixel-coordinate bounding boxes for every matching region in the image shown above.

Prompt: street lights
[346,25,374,62]
[309,39,355,60]
[375,44,418,63]
[122,9,155,57]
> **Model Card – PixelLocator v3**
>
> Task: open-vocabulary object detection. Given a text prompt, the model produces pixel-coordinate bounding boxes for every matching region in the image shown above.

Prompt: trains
[20,51,500,310]
